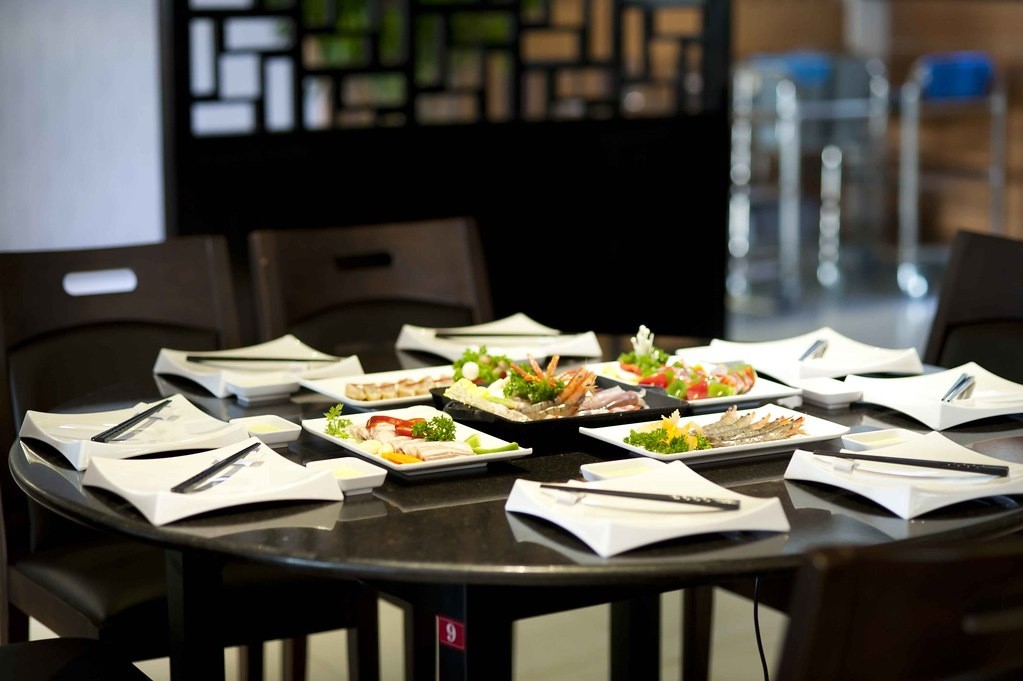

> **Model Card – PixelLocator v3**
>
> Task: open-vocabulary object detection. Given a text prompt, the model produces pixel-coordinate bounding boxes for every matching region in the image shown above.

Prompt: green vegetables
[620,349,670,375]
[411,415,457,441]
[453,346,567,402]
[323,403,354,440]
[624,427,712,455]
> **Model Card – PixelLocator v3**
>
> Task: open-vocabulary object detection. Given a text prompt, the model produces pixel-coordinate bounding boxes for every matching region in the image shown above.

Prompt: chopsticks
[91,400,171,442]
[170,443,261,492]
[436,333,554,340]
[187,354,337,364]
[942,373,975,401]
[799,339,824,362]
[813,450,1009,477]
[540,484,739,510]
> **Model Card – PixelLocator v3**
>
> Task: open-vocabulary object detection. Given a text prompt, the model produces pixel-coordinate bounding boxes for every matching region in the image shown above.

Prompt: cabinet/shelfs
[729,52,1006,296]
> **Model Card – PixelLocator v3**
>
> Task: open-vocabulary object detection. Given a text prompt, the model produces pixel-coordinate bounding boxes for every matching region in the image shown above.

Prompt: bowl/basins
[228,377,299,402]
[305,457,387,495]
[841,427,923,451]
[580,457,667,480]
[230,413,301,448]
[788,376,862,410]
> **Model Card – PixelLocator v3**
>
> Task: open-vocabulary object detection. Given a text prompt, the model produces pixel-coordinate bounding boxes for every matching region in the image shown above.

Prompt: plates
[429,355,689,442]
[846,361,1023,432]
[578,403,851,465]
[785,431,1023,520]
[583,354,802,413]
[302,405,532,475]
[154,333,364,399]
[81,437,343,524]
[395,313,604,363]
[505,459,786,556]
[712,326,924,387]
[20,394,249,471]
[298,365,455,412]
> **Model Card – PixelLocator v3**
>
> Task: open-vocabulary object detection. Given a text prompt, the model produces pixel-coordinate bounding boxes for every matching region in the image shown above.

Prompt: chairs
[0,215,1023,681]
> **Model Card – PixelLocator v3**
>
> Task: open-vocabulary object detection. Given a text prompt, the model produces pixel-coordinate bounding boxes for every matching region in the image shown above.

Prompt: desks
[8,334,1022,681]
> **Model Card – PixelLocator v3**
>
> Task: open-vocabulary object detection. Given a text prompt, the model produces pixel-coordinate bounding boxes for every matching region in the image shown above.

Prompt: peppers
[382,452,423,463]
[637,362,755,401]
[366,415,429,439]
[464,434,519,453]
